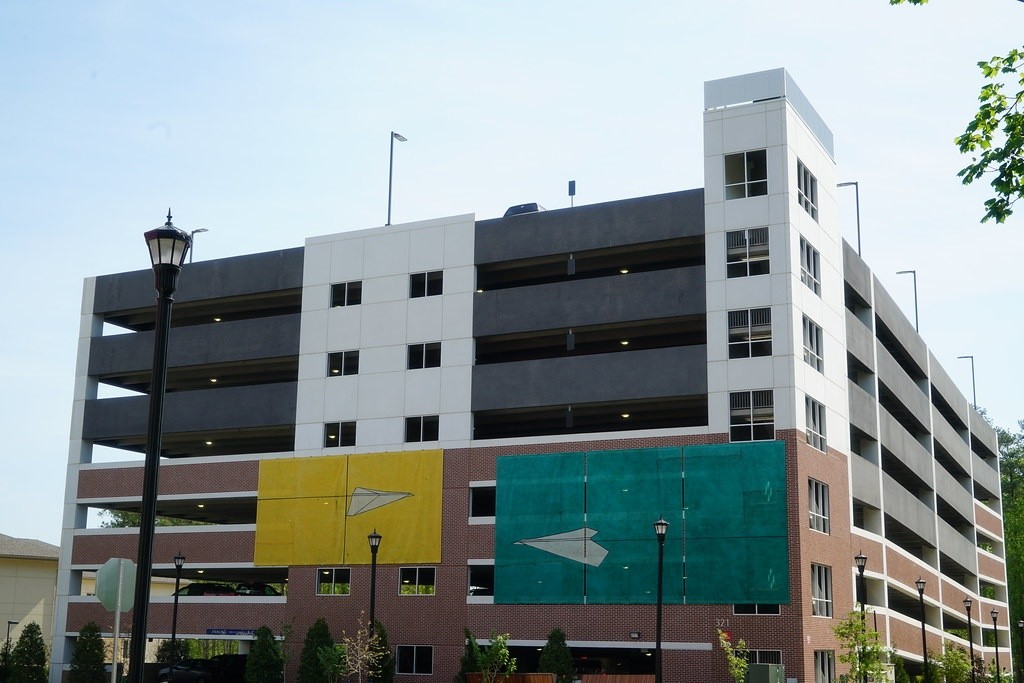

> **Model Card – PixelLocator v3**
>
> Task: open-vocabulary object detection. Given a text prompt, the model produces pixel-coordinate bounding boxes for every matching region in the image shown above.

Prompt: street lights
[915,576,929,683]
[837,182,861,257]
[190,227,209,263]
[854,549,867,683]
[991,607,1000,683]
[7,620,19,652]
[957,356,977,412]
[653,516,669,683]
[368,528,382,670]
[169,551,186,683]
[963,595,975,683]
[129,208,192,683]
[387,131,407,226]
[895,270,918,335]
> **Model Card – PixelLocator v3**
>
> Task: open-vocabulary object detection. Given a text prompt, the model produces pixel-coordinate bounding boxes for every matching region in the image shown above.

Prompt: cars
[502,203,547,217]
[172,583,281,596]
[159,654,248,683]
